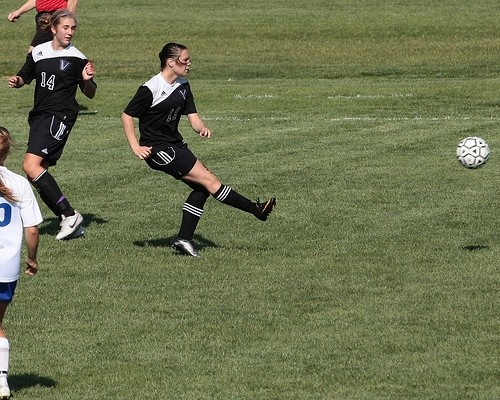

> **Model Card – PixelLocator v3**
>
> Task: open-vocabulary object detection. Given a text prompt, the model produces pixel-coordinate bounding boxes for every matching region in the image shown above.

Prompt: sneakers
[171,237,201,258]
[253,197,276,221]
[0,382,12,399]
[55,210,84,240]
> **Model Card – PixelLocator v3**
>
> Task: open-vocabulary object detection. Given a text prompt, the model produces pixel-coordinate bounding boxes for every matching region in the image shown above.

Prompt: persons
[8,0,78,63]
[0,125,44,400]
[8,9,98,242]
[121,42,276,258]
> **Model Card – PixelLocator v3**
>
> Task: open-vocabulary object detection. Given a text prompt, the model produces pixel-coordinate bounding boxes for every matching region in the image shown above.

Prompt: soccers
[455,136,492,170]
